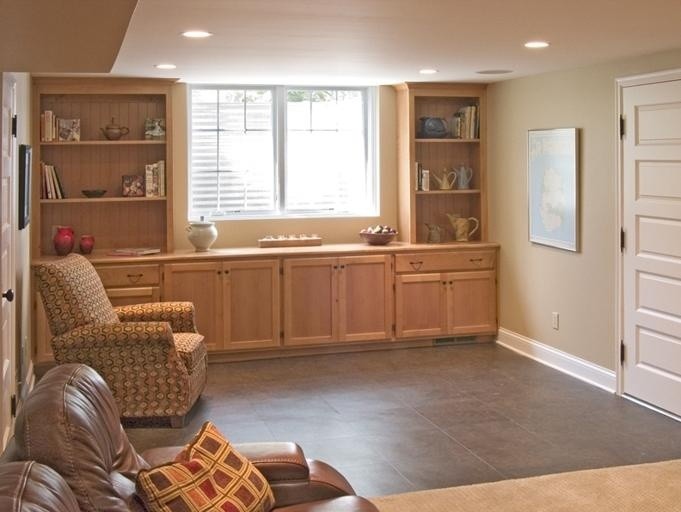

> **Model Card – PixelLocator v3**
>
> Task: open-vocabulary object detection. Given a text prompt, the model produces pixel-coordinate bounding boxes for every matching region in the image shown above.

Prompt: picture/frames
[19,144,32,230]
[527,127,580,253]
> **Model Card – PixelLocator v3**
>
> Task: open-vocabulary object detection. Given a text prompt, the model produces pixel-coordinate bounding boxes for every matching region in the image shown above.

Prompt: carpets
[367,456,681,512]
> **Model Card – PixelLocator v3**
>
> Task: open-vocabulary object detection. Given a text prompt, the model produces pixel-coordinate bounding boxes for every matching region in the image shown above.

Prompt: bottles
[452,113,463,138]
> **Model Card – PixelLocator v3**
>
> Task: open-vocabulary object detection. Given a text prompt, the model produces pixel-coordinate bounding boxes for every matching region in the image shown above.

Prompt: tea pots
[99,115,131,140]
[451,164,474,191]
[423,222,447,244]
[446,211,481,243]
[431,167,457,190]
[419,115,450,138]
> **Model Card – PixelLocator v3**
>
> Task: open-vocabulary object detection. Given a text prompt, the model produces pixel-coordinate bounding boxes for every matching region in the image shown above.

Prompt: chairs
[31,253,207,427]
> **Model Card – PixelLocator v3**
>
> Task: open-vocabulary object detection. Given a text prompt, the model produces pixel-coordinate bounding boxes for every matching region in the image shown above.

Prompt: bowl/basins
[359,231,400,245]
[81,189,107,198]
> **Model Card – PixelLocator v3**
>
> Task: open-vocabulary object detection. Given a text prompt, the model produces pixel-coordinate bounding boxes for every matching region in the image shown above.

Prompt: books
[40,109,59,142]
[450,104,480,139]
[416,161,429,191]
[40,160,66,199]
[107,247,160,256]
[144,160,165,197]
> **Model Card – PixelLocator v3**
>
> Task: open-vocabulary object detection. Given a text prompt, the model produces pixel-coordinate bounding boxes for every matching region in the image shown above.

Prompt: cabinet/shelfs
[394,82,488,243]
[161,257,282,363]
[36,261,161,363]
[282,252,395,358]
[395,244,501,348]
[29,74,178,252]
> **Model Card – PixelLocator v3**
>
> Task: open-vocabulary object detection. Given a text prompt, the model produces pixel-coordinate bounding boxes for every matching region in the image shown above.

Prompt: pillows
[135,421,276,512]
